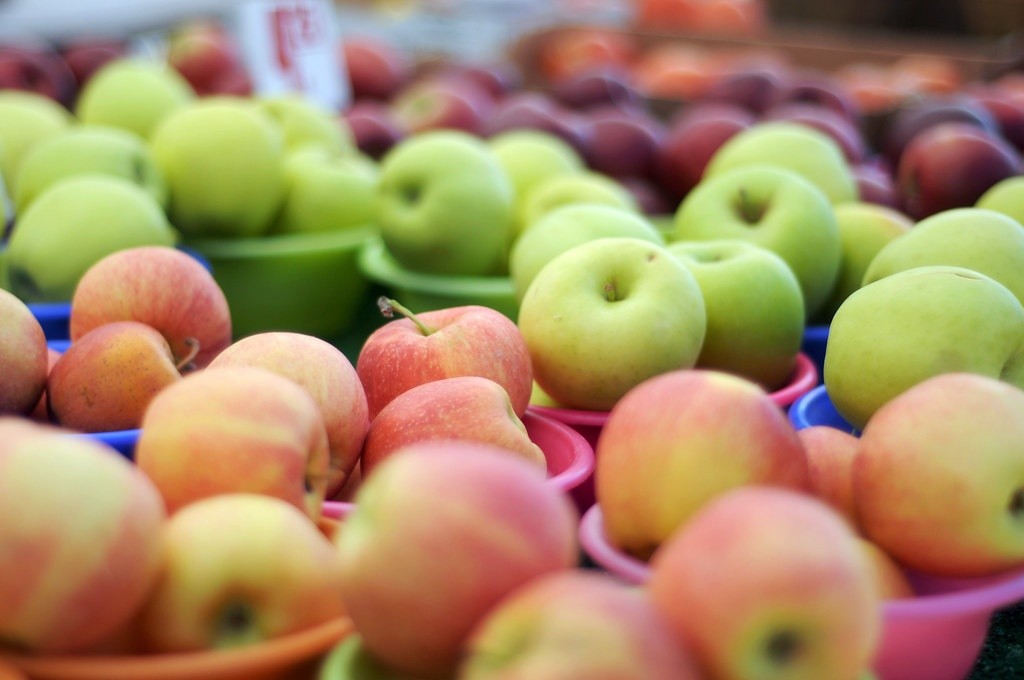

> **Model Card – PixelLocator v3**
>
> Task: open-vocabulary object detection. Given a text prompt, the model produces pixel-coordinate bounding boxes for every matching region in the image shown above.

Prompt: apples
[0,25,1024,680]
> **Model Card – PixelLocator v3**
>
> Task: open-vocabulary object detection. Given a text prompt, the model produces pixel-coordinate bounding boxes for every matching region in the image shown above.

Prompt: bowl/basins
[0,232,1024,680]
